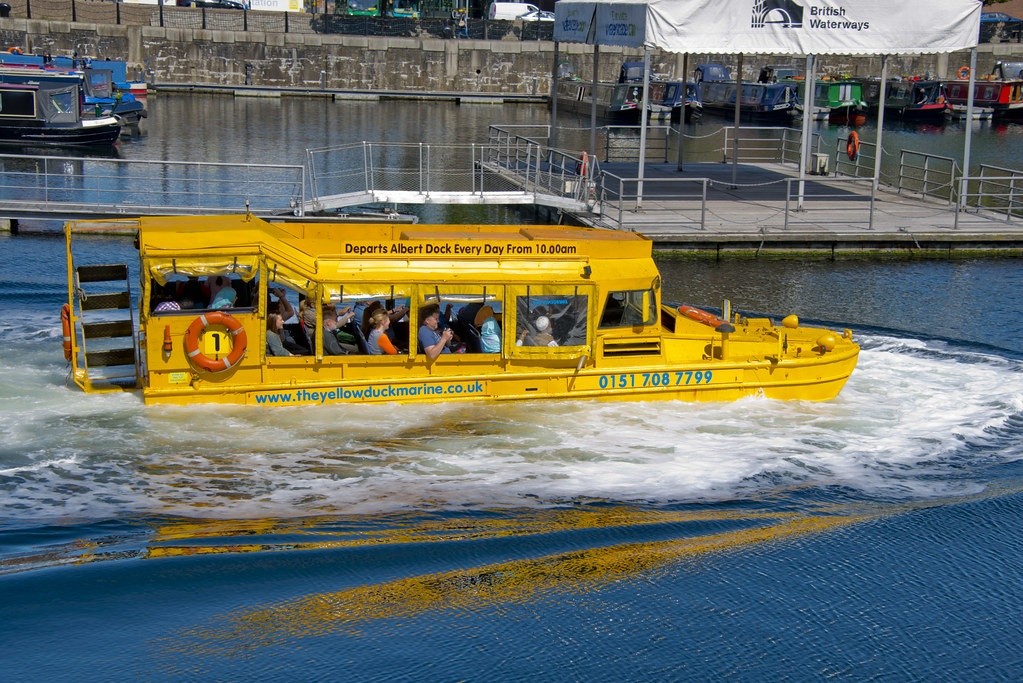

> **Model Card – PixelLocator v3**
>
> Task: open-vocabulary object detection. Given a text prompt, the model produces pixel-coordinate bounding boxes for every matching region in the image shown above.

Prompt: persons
[151,276,238,311]
[516,306,559,346]
[417,302,502,361]
[251,280,301,356]
[298,295,410,356]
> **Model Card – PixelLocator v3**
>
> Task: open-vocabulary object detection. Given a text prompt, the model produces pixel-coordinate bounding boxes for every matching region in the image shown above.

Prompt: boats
[1,46,150,153]
[692,64,798,115]
[551,76,653,122]
[759,65,863,112]
[618,63,703,115]
[944,78,1023,110]
[59,200,860,404]
[832,74,950,114]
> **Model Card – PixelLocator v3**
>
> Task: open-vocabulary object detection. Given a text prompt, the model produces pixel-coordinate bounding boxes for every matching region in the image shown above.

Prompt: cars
[175,0,243,10]
[516,11,555,21]
[981,12,1021,23]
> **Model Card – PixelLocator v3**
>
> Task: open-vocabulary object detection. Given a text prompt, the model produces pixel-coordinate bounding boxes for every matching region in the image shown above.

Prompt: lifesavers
[678,305,729,327]
[847,130,859,160]
[794,76,805,80]
[8,46,23,54]
[61,303,72,363]
[184,312,247,373]
[822,76,836,82]
[957,66,970,80]
[580,151,589,176]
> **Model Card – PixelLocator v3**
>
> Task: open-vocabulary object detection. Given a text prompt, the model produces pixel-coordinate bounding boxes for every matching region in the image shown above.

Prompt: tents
[546,0,983,214]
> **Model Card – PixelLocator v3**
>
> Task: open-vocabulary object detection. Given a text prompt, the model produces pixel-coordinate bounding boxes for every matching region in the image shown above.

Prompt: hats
[535,316,550,331]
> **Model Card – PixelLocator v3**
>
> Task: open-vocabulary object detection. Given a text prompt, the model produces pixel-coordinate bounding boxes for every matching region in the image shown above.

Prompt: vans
[488,2,541,20]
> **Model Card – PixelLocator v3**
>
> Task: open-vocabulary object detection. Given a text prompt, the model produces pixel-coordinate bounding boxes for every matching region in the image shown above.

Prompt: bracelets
[392,309,395,313]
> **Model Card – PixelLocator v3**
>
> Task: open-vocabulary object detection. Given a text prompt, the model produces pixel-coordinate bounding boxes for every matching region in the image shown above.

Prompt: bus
[383,0,425,19]
[334,0,381,18]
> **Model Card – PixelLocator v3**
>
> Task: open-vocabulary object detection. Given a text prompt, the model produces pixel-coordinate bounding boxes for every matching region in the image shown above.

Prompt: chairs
[264,307,538,358]
